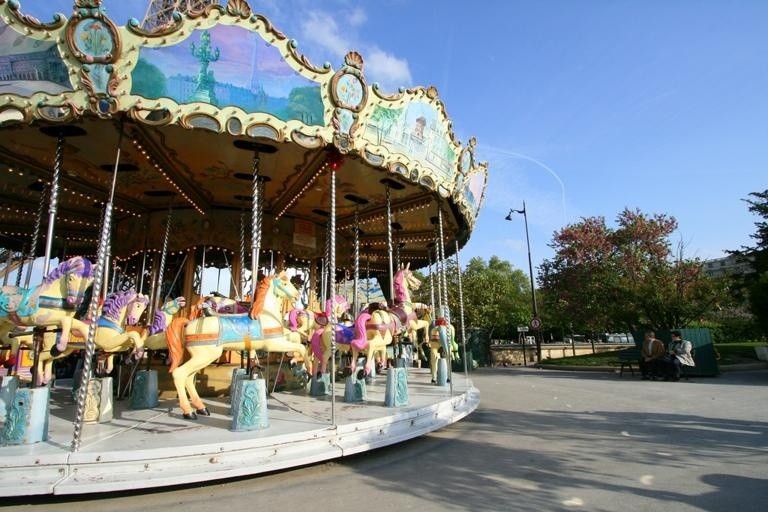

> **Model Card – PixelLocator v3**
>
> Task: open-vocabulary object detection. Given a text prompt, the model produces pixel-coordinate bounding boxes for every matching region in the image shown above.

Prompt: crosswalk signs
[530,317,541,331]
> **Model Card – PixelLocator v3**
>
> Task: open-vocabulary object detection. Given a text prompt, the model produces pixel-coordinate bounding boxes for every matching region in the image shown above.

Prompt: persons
[638,331,695,381]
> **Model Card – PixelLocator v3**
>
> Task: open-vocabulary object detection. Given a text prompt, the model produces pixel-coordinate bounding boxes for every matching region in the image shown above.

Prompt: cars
[521,335,535,345]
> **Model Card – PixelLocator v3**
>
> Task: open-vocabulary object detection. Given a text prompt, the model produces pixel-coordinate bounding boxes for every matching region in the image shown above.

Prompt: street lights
[505,199,542,364]
